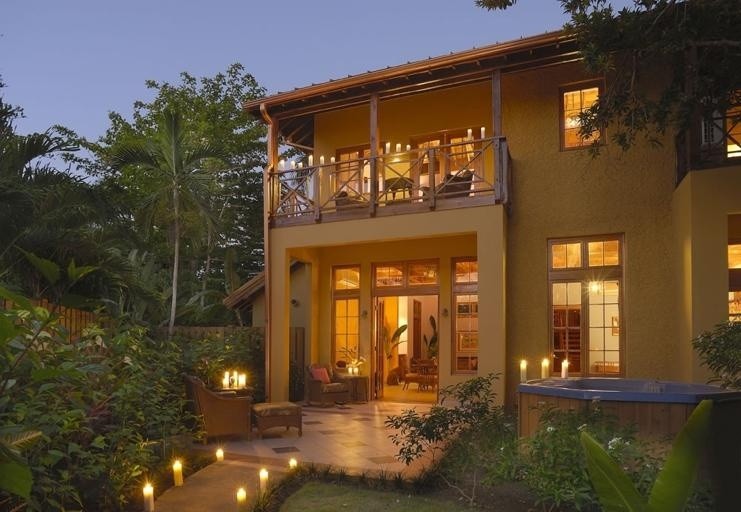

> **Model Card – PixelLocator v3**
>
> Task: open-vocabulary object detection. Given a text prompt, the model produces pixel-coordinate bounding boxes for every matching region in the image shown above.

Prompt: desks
[213,384,255,397]
[334,374,368,404]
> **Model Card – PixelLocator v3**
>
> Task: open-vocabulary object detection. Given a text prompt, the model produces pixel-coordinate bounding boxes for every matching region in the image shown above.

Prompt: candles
[541,359,549,379]
[348,367,352,375]
[612,315,620,336]
[562,360,568,379]
[481,127,485,138]
[467,128,472,141]
[520,360,527,383]
[354,367,358,375]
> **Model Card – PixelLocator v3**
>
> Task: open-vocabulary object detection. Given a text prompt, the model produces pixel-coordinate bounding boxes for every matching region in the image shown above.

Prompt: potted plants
[383,325,407,385]
[339,344,369,373]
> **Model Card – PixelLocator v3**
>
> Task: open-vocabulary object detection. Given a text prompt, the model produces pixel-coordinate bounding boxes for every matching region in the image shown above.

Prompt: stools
[252,401,303,440]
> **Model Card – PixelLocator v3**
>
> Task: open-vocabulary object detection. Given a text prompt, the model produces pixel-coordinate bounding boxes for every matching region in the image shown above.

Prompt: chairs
[399,359,439,393]
[422,170,475,201]
[335,191,366,212]
[306,363,349,408]
[185,375,253,445]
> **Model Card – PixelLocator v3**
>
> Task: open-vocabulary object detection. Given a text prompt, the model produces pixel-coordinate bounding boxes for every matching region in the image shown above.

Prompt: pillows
[311,367,330,384]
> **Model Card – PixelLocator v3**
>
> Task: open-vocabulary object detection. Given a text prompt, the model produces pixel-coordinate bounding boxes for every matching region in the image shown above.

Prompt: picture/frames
[458,330,479,351]
[457,304,472,318]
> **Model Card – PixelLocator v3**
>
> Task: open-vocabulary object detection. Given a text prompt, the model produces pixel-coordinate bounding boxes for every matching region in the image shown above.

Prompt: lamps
[142,481,155,511]
[291,161,295,168]
[237,487,247,512]
[396,144,402,152]
[330,157,336,163]
[222,370,246,388]
[173,461,184,487]
[407,145,411,152]
[290,458,297,471]
[298,162,302,168]
[216,449,223,462]
[320,156,324,165]
[309,156,313,167]
[281,160,284,169]
[385,142,391,153]
[260,468,268,494]
